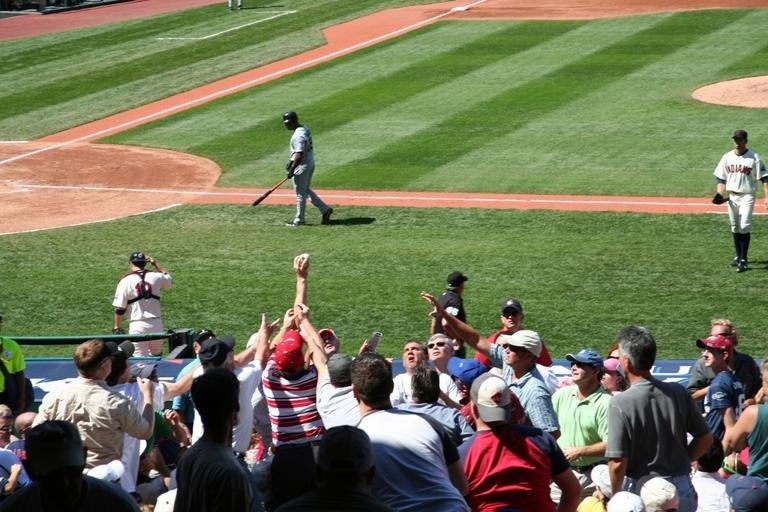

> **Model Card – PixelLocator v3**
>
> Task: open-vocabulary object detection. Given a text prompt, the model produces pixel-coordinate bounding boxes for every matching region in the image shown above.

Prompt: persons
[111,252,173,361]
[0,417,33,495]
[283,112,333,227]
[695,335,747,471]
[604,325,714,512]
[271,424,397,512]
[227,0,243,8]
[0,404,19,449]
[550,349,613,497]
[712,130,768,272]
[297,309,367,431]
[30,338,155,512]
[687,318,764,417]
[0,420,142,512]
[102,339,198,501]
[431,271,468,359]
[137,421,191,504]
[426,334,465,411]
[128,363,191,486]
[454,372,582,512]
[395,366,475,449]
[173,368,265,512]
[172,323,278,451]
[262,308,325,510]
[473,297,552,372]
[293,254,340,362]
[725,359,768,486]
[7,411,38,485]
[420,291,561,442]
[387,339,464,408]
[190,314,281,512]
[576,463,629,512]
[0,315,25,413]
[608,347,623,361]
[351,348,472,511]
[0,463,23,502]
[600,357,626,391]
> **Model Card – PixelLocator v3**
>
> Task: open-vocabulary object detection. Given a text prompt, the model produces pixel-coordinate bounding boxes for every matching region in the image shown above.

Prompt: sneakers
[728,256,738,265]
[284,221,304,227]
[736,259,746,272]
[321,208,332,224]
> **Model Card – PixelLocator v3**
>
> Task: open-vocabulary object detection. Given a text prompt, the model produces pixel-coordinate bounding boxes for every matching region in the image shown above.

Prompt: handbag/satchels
[5,374,33,412]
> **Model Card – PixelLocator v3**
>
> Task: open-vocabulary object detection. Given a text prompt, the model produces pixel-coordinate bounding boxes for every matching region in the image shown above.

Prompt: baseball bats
[252,176,290,206]
[717,197,730,203]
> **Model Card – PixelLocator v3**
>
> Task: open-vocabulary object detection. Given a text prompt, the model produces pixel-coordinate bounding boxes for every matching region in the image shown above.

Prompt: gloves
[287,167,293,178]
[285,160,293,169]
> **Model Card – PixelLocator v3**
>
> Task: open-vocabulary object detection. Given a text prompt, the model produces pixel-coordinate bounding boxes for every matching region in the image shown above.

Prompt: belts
[570,463,598,474]
[274,441,321,448]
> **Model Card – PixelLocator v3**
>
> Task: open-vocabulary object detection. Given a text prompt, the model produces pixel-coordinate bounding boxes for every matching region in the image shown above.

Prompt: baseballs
[299,254,310,269]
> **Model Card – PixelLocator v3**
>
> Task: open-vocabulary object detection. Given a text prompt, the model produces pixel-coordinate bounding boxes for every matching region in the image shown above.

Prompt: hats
[328,353,356,372]
[274,331,303,371]
[446,356,488,388]
[317,425,372,478]
[500,330,541,359]
[731,130,746,138]
[601,358,625,379]
[104,340,134,372]
[564,349,604,375]
[501,298,521,313]
[193,329,215,341]
[470,373,511,423]
[24,421,84,486]
[446,271,467,286]
[590,464,628,500]
[77,341,117,369]
[128,362,157,382]
[198,336,234,367]
[695,335,732,359]
[317,328,337,339]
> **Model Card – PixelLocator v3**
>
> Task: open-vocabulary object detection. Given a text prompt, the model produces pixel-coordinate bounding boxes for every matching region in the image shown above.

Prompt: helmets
[283,111,296,123]
[129,252,145,262]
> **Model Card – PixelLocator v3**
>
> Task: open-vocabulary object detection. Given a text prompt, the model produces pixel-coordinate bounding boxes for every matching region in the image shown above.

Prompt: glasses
[502,310,517,316]
[426,342,444,348]
[502,344,524,352]
